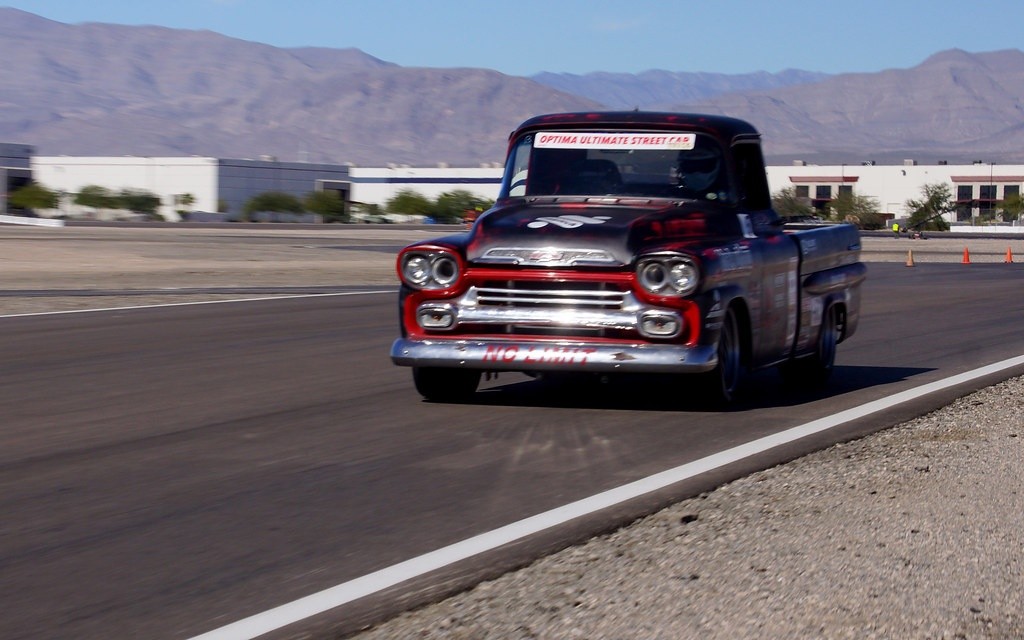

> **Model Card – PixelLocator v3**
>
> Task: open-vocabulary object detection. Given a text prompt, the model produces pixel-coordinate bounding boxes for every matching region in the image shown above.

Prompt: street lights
[987,162,996,225]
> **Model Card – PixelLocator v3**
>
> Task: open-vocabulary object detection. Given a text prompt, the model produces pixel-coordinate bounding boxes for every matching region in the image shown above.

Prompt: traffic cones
[1003,245,1014,264]
[906,249,918,268]
[960,244,973,264]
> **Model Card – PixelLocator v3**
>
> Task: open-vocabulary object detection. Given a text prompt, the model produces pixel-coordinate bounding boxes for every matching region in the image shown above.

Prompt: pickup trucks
[390,109,870,410]
